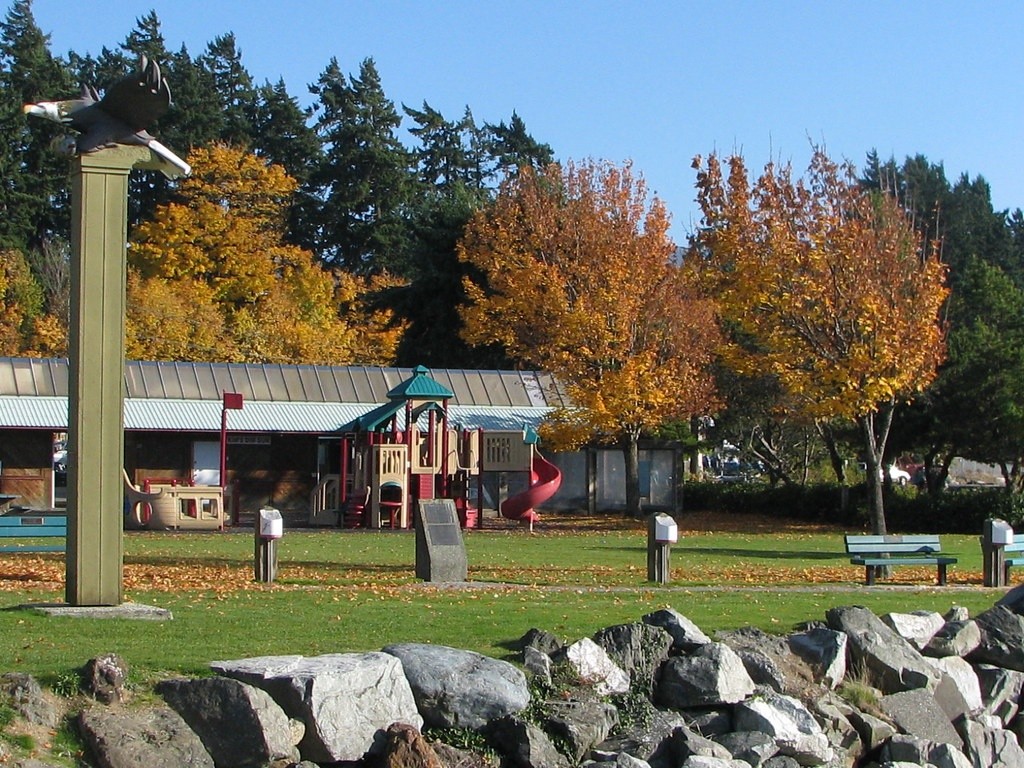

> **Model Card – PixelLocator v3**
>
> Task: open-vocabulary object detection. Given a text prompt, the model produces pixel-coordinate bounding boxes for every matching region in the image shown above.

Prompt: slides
[500,454,563,525]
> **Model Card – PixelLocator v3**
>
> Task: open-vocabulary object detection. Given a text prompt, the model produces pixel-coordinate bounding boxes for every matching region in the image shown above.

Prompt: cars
[879,462,912,487]
[701,445,765,475]
[910,462,952,487]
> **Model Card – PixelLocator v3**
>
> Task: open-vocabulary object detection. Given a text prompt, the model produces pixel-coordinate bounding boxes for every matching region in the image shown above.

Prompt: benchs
[979,534,1024,586]
[844,535,957,585]
